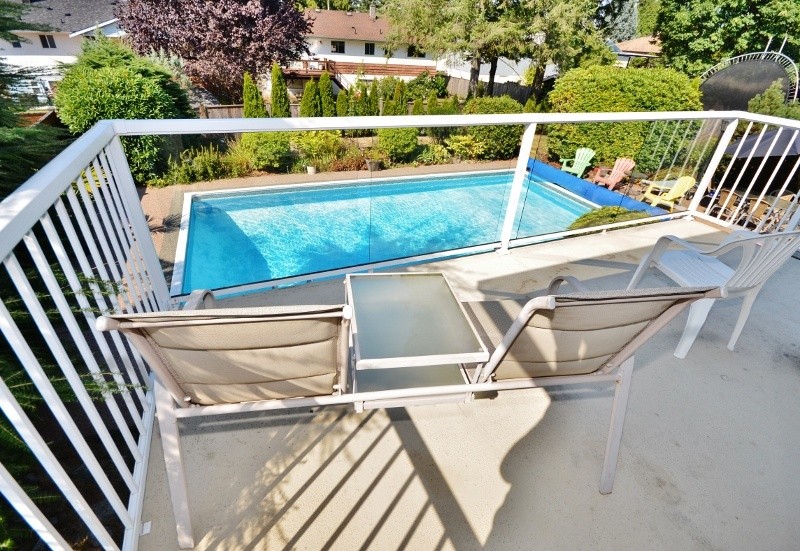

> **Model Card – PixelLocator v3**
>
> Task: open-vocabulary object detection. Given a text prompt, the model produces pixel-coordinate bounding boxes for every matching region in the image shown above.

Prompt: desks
[344,272,489,409]
[760,196,799,211]
[725,190,759,207]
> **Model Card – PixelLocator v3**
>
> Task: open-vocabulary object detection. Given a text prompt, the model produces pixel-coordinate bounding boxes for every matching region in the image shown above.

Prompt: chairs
[559,147,596,179]
[95,230,800,550]
[640,175,696,212]
[594,157,635,190]
[709,188,797,229]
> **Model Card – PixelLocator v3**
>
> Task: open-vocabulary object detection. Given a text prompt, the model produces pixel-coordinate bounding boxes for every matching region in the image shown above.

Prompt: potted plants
[364,150,380,171]
[307,160,317,174]
